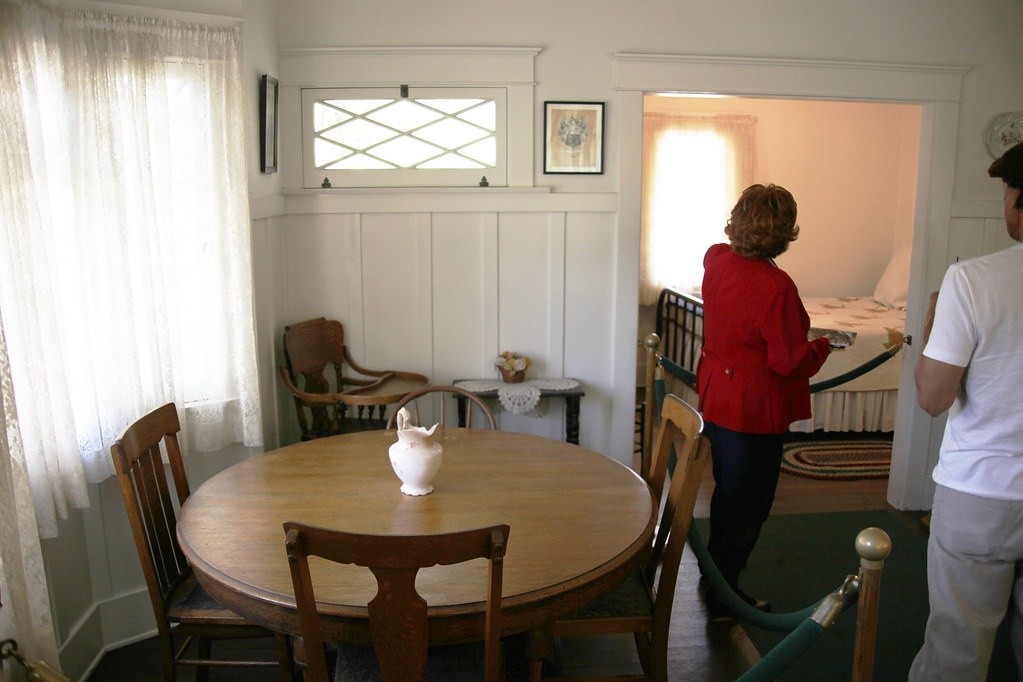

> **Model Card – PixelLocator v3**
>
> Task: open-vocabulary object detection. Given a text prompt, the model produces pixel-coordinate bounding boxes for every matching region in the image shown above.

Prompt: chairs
[110,402,295,682]
[281,317,430,443]
[522,393,717,682]
[282,519,513,682]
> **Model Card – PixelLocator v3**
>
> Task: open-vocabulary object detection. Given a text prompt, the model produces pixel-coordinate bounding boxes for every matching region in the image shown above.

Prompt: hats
[988,140,1023,182]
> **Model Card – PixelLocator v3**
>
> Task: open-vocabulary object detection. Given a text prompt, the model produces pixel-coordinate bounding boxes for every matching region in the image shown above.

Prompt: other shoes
[704,581,773,618]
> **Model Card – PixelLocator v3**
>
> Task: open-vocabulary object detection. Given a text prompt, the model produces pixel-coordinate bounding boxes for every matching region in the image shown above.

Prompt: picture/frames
[258,73,279,171]
[544,101,604,174]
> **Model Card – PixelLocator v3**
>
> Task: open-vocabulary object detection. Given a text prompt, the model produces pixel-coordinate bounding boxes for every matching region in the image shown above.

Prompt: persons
[697,184,832,620]
[907,142,1023,682]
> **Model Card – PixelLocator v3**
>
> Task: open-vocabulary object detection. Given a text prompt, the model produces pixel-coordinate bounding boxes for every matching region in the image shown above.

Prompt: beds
[652,289,911,434]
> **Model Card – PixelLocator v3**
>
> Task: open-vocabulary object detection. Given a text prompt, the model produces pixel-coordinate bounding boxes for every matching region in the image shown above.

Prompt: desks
[172,427,653,682]
[453,378,586,445]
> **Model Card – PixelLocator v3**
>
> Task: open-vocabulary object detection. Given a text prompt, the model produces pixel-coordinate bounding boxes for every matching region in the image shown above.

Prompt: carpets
[781,437,894,480]
[689,509,1023,682]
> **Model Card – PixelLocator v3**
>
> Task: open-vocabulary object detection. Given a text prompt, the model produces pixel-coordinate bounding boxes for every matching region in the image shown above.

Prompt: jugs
[388,406,444,496]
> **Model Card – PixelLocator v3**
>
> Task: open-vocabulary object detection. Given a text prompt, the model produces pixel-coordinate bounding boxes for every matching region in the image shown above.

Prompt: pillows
[874,241,915,308]
